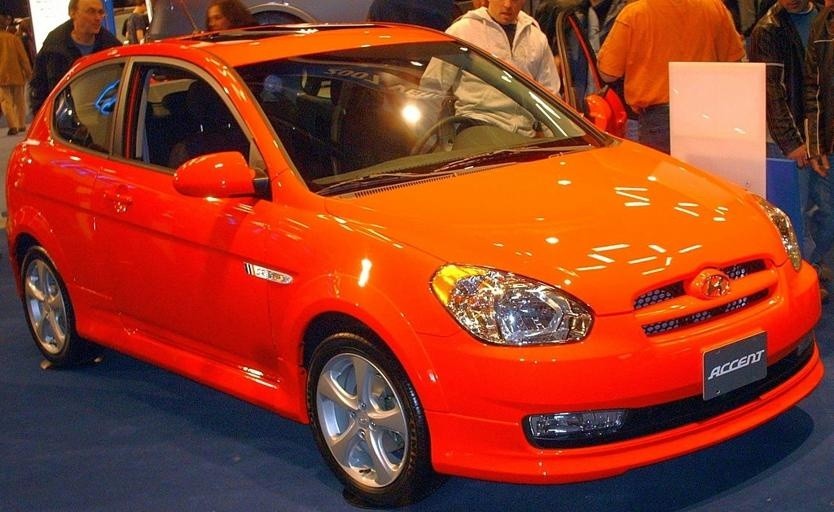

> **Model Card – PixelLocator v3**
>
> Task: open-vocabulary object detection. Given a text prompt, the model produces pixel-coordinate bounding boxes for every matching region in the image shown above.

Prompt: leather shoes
[7,127,26,134]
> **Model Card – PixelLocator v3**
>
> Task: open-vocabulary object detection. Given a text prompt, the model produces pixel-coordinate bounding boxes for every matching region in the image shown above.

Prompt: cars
[4,10,826,508]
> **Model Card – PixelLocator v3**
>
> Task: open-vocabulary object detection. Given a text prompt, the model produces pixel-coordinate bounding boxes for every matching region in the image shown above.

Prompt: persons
[368,0,453,33]
[407,0,563,151]
[520,1,834,301]
[0,13,37,136]
[125,0,147,44]
[205,1,257,32]
[24,0,124,117]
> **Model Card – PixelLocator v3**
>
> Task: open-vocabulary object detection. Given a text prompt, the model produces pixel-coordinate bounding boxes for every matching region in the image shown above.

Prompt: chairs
[330,71,417,177]
[169,80,250,170]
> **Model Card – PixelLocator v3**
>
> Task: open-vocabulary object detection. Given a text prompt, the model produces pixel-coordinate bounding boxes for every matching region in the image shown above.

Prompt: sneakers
[819,281,833,304]
